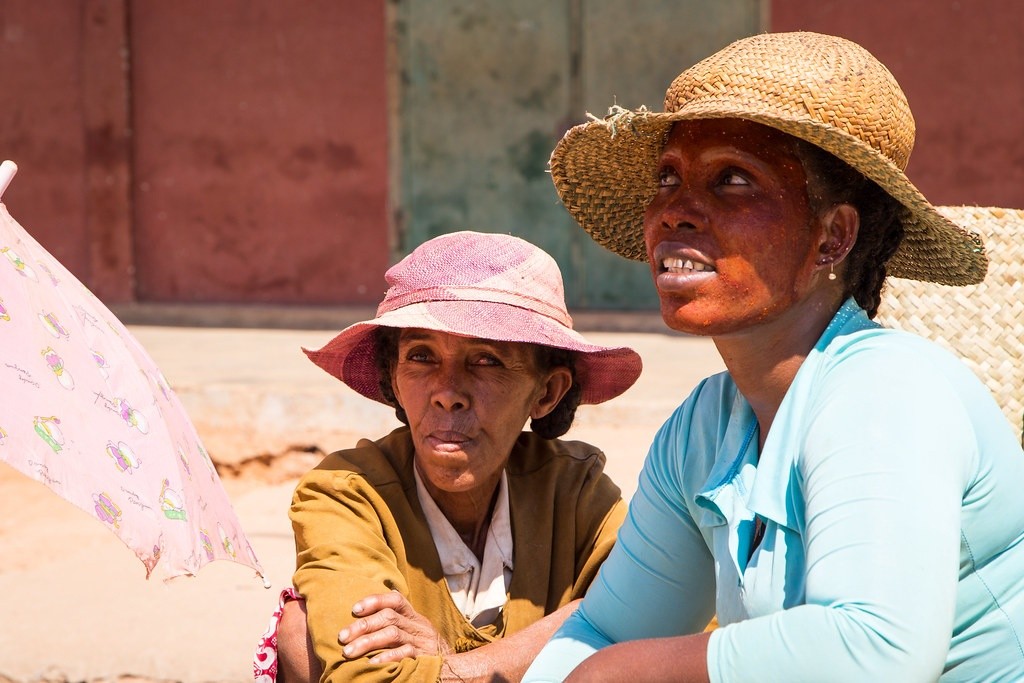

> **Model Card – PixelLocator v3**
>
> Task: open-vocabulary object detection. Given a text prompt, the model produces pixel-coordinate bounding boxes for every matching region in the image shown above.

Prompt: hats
[548,31,988,288]
[299,229,642,406]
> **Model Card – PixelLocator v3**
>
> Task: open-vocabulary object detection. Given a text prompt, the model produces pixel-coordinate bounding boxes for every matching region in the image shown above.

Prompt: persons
[522,30,1024,682]
[277,231,719,683]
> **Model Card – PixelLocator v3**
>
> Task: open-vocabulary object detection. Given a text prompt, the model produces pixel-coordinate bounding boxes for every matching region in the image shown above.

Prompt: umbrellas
[1,158,272,592]
